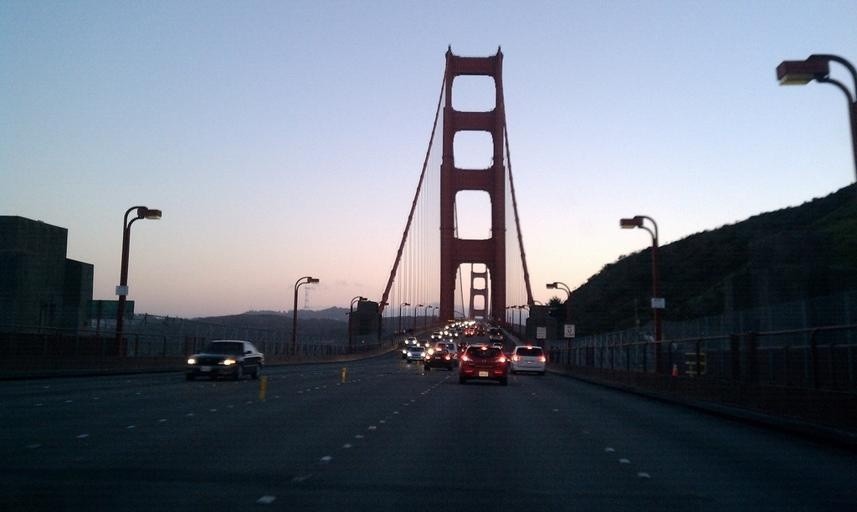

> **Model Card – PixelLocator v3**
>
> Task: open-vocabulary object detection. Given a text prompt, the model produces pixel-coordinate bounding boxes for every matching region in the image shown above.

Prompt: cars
[402,342,457,371]
[459,343,510,386]
[511,345,546,374]
[186,339,266,380]
[431,320,488,340]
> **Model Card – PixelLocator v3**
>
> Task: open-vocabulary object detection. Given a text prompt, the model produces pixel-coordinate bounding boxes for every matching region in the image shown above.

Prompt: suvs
[487,327,504,342]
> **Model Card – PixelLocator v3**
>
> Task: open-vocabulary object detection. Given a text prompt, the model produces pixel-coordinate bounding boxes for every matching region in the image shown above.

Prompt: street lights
[117,205,163,356]
[505,305,524,335]
[776,54,856,183]
[292,277,319,353]
[348,296,438,348]
[620,215,660,373]
[546,282,572,365]
